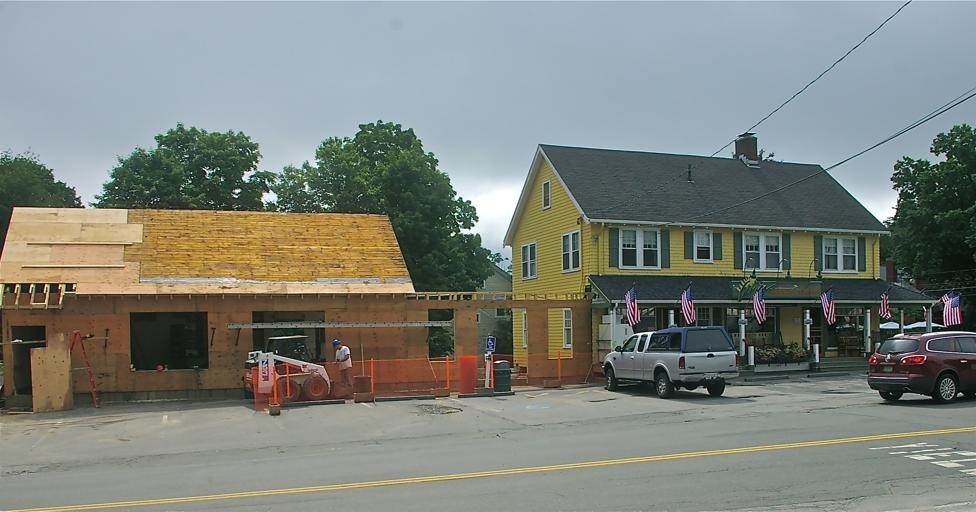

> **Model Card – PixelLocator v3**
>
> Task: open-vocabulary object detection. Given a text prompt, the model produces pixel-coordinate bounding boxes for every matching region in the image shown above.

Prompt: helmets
[333,339,340,345]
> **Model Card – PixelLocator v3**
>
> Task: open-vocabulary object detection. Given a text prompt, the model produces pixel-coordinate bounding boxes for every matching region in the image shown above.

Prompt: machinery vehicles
[242,335,332,403]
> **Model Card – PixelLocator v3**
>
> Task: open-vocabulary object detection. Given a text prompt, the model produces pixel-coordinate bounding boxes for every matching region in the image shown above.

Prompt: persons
[331,338,355,388]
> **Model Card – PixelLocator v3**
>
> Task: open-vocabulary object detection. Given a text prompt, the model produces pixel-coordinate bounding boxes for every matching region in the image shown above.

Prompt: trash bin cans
[494,360,511,392]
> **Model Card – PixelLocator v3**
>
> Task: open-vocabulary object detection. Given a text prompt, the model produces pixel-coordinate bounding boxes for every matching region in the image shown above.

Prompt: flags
[817,286,837,325]
[680,284,696,325]
[623,286,641,326]
[943,296,963,328]
[941,289,956,304]
[876,288,892,320]
[751,287,766,326]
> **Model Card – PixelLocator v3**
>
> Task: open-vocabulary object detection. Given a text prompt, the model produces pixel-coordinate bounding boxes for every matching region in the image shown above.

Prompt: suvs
[865,329,976,405]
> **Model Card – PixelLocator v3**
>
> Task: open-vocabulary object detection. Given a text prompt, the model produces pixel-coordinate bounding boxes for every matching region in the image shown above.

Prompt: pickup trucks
[600,324,743,399]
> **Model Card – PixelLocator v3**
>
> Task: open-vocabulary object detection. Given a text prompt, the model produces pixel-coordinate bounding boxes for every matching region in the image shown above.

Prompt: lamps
[744,257,823,282]
